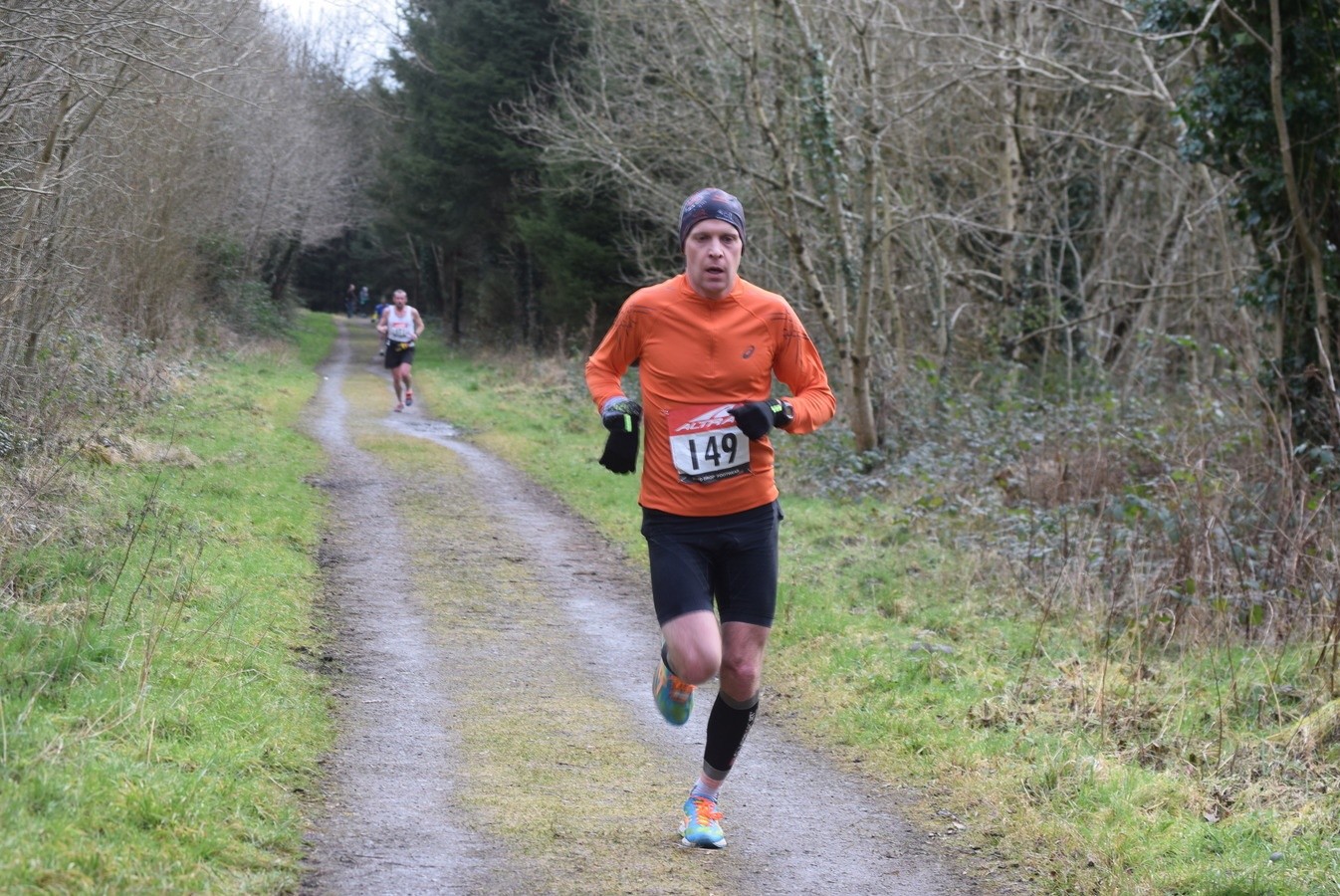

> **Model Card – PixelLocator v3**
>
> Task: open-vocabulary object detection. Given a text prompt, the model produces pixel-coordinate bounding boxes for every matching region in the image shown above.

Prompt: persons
[582,186,838,856]
[345,284,358,317]
[372,294,389,354]
[378,288,424,415]
[359,287,369,317]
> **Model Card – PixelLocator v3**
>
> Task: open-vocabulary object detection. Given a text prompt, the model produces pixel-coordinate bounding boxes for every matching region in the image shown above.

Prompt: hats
[678,187,744,257]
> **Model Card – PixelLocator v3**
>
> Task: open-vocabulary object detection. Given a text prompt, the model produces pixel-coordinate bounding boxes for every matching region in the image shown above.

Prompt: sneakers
[678,787,726,848]
[651,630,690,726]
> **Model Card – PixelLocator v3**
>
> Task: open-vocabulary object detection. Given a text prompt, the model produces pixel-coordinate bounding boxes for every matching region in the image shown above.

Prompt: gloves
[727,398,785,439]
[602,397,642,434]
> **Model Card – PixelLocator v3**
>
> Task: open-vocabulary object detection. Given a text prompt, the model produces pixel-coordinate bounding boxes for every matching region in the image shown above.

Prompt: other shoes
[395,402,405,413]
[406,392,412,406]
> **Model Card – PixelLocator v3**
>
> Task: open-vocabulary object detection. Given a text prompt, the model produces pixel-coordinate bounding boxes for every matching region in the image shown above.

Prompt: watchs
[781,400,796,428]
